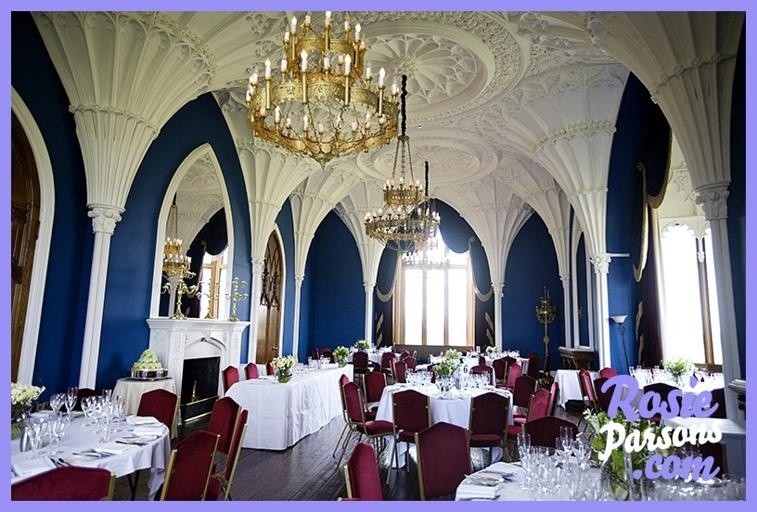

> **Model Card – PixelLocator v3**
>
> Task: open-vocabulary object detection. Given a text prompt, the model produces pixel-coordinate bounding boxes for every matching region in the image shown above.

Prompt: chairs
[224,344,333,388]
[10,388,249,501]
[333,345,725,502]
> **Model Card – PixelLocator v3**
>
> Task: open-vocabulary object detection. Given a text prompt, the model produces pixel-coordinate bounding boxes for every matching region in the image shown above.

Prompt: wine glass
[20,387,126,459]
[404,368,490,395]
[516,425,611,502]
[293,354,330,378]
[628,364,664,387]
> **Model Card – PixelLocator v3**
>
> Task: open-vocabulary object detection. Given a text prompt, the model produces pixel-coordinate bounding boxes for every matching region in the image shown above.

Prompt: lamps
[161,256,197,319]
[244,11,402,166]
[610,315,630,375]
[162,191,192,278]
[535,286,558,369]
[225,276,249,321]
[363,74,452,272]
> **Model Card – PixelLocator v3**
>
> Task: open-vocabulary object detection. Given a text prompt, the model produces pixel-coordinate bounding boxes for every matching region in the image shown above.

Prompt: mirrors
[150,141,235,318]
